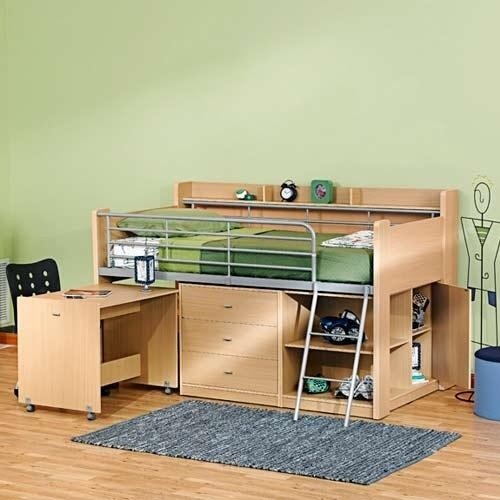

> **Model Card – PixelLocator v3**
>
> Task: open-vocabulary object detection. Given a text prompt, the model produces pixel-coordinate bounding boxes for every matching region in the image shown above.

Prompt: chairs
[6,258,61,398]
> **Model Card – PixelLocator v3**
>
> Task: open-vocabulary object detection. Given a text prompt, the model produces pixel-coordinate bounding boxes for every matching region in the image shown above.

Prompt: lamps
[134,248,156,292]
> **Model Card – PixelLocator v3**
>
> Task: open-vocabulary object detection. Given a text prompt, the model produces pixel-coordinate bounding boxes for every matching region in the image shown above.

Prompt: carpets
[70,400,462,485]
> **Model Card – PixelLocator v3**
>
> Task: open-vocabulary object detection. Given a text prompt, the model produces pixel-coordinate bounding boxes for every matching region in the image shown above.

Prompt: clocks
[280,179,298,202]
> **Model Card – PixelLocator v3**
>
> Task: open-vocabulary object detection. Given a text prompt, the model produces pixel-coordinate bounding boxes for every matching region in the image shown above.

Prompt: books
[63,289,112,298]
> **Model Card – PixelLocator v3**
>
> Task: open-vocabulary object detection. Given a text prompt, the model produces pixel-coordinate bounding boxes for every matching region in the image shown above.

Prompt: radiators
[0,257,10,325]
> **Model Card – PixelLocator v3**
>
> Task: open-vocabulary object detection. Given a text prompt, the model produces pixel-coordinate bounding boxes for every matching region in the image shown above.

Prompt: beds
[92,181,460,295]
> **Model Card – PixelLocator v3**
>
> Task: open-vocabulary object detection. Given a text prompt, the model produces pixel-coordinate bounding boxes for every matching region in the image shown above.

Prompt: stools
[473,345,500,421]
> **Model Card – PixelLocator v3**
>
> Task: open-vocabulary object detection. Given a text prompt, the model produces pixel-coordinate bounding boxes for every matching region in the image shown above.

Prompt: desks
[17,284,179,421]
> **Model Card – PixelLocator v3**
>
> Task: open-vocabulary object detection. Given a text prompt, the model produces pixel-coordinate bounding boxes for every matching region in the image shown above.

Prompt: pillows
[116,207,243,238]
